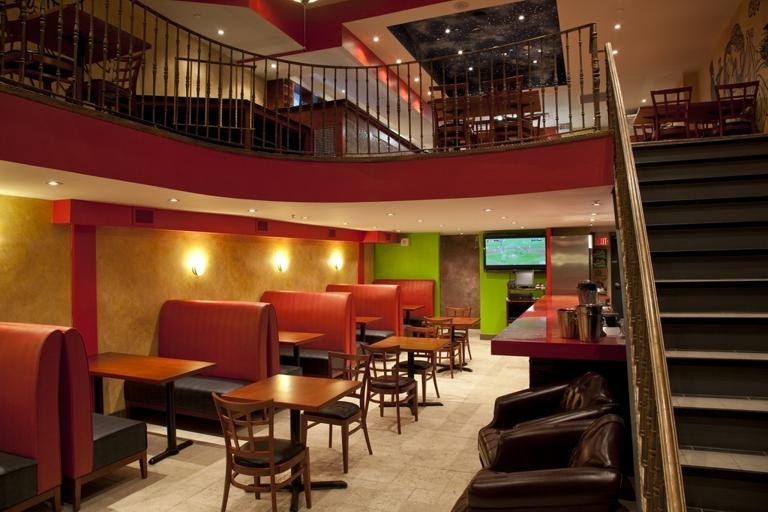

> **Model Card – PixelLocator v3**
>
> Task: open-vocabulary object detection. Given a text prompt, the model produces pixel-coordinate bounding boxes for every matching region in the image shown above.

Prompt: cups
[557,278,619,343]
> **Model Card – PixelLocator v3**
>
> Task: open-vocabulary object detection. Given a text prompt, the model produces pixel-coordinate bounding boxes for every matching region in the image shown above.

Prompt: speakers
[401,239,410,246]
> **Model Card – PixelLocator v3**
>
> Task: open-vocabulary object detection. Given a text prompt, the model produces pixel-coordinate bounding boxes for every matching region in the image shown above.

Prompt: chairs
[0,0,146,118]
[391,306,473,404]
[453,369,630,512]
[212,391,312,512]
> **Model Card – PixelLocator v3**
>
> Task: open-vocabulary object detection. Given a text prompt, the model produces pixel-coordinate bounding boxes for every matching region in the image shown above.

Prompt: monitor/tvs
[483,233,546,273]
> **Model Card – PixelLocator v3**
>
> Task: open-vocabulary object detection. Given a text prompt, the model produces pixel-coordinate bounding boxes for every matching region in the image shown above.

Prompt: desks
[422,316,482,377]
[136,94,315,154]
